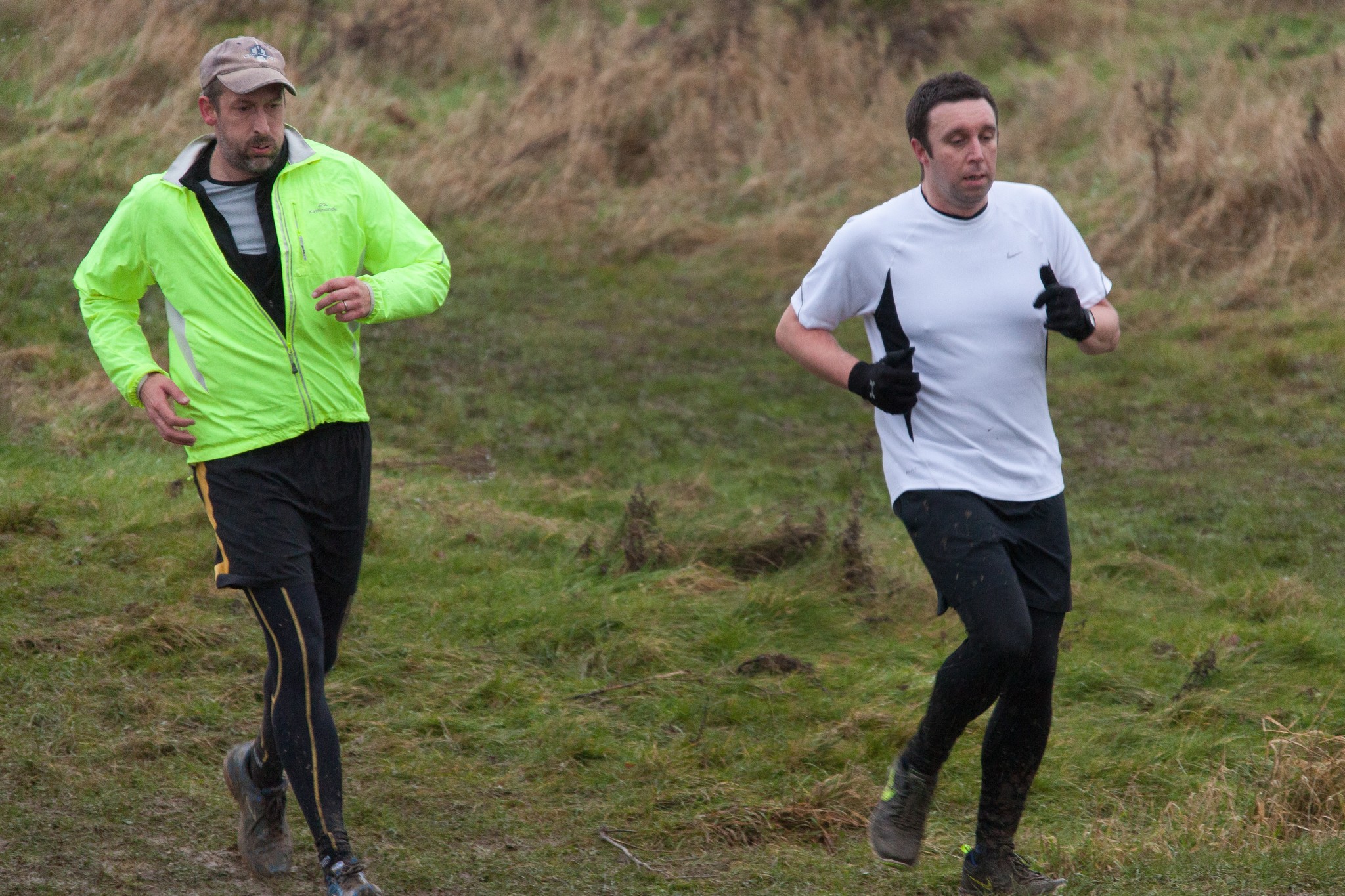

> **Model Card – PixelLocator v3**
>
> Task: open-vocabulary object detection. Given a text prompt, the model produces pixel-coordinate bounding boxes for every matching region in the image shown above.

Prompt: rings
[342,300,348,311]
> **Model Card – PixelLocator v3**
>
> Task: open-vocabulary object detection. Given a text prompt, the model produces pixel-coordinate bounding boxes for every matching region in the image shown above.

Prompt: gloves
[1032,265,1096,343]
[847,346,922,414]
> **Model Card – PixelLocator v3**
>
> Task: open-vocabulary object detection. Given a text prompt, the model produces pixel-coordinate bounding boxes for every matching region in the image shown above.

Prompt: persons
[70,36,454,896]
[773,72,1127,896]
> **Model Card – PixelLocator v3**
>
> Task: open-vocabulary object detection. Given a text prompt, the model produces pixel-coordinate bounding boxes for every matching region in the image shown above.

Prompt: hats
[199,36,297,97]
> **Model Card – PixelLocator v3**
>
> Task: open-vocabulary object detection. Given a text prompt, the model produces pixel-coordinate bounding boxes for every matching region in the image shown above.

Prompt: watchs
[1076,308,1097,342]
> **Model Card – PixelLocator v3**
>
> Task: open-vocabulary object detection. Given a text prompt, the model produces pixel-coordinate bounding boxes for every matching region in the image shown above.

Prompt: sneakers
[325,857,382,896]
[868,760,938,869]
[223,740,294,880]
[960,843,1067,895]
